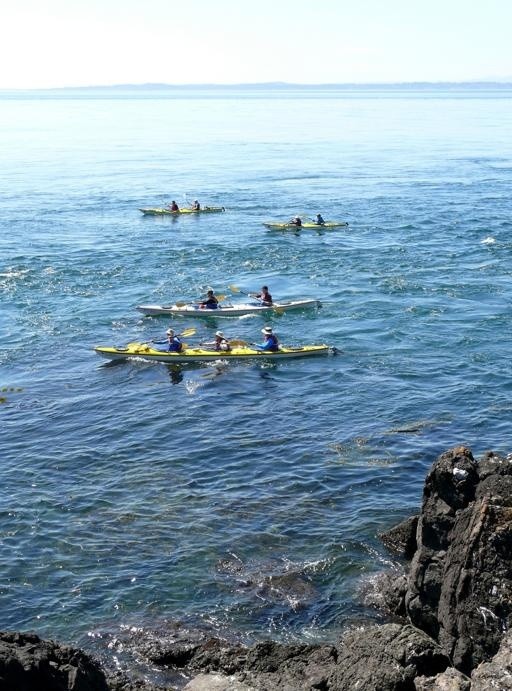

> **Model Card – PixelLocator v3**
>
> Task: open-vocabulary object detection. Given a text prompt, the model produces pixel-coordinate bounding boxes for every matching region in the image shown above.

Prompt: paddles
[181,340,248,348]
[176,295,225,307]
[231,287,284,315]
[140,329,196,345]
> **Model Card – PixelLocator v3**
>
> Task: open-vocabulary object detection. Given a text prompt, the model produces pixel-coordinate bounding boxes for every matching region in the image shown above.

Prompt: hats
[166,329,175,335]
[261,327,273,335]
[216,331,225,339]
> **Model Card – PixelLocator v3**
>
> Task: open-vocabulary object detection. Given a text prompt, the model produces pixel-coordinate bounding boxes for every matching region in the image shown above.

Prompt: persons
[151,327,183,350]
[166,199,178,210]
[248,285,272,306]
[201,360,227,380]
[198,330,231,352]
[308,212,325,224]
[190,198,200,210]
[190,286,219,308]
[257,361,277,383]
[250,325,280,352]
[289,214,301,226]
[165,362,184,386]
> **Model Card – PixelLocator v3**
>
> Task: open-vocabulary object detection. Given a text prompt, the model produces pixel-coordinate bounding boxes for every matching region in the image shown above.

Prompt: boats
[96,356,336,372]
[143,214,222,223]
[145,310,321,325]
[136,299,323,317]
[267,229,346,235]
[261,221,346,230]
[139,207,222,216]
[95,342,329,362]
